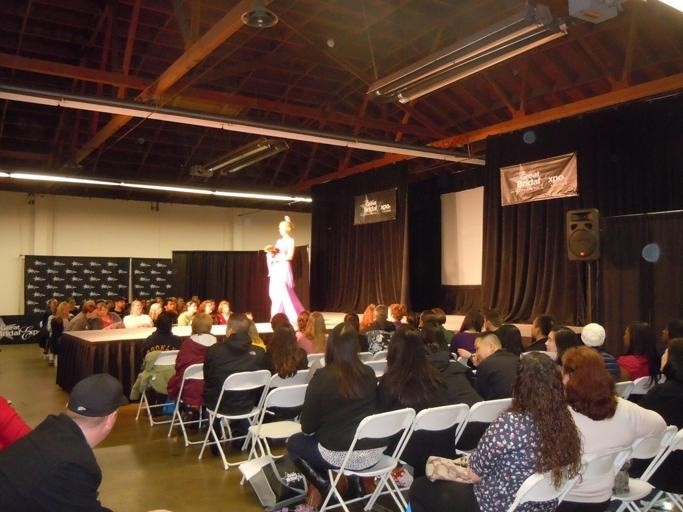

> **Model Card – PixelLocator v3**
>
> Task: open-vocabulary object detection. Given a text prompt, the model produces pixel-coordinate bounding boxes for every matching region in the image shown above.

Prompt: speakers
[567,208,611,262]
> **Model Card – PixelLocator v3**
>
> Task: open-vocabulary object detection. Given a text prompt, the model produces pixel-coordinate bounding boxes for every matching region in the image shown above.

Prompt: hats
[69,374,130,417]
[581,322,607,347]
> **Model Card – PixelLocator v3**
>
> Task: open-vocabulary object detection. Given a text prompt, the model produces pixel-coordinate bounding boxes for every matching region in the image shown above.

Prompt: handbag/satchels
[426,454,480,484]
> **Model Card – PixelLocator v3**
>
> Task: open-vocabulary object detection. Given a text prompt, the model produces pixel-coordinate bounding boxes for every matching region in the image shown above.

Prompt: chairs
[611,381,635,401]
[135,348,520,512]
[618,431,682,512]
[606,424,678,512]
[510,461,589,512]
[635,374,665,396]
[566,446,632,511]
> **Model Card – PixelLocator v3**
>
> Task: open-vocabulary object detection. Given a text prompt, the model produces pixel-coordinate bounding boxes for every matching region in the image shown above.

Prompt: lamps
[363,4,570,108]
[189,136,291,179]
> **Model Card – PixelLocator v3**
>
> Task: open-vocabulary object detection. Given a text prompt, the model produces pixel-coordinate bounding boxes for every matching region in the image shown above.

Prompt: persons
[41,296,255,366]
[274,216,305,315]
[0,372,169,512]
[142,303,683,512]
